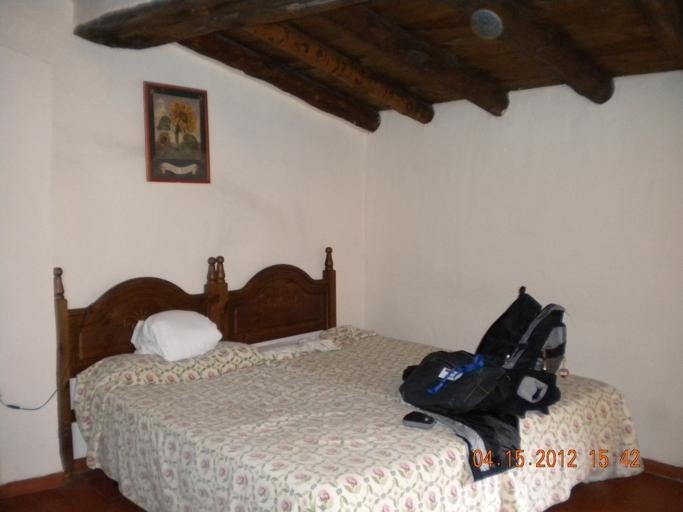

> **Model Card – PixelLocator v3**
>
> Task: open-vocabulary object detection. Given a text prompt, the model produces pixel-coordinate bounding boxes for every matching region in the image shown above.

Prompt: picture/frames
[143,80,210,184]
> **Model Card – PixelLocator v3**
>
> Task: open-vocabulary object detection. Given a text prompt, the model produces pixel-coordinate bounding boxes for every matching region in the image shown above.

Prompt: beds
[54,246,646,511]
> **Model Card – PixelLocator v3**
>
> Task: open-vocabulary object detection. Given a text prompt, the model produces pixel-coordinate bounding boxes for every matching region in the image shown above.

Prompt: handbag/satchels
[399,350,516,421]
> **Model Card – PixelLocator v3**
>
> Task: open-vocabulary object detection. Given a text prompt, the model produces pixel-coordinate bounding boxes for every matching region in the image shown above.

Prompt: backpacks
[476,286,566,374]
